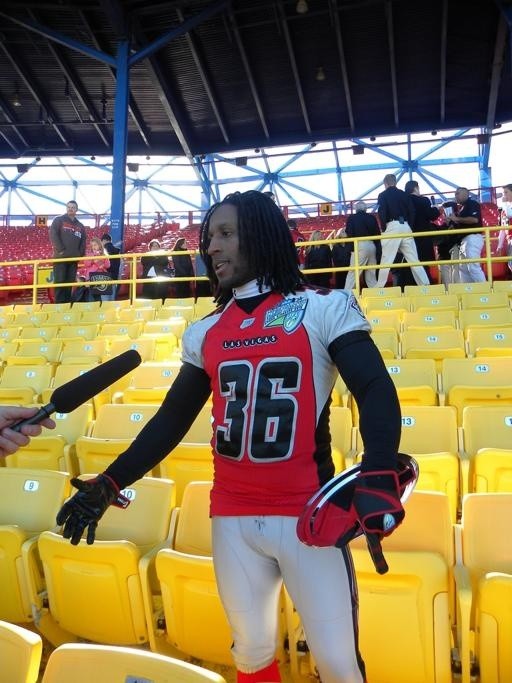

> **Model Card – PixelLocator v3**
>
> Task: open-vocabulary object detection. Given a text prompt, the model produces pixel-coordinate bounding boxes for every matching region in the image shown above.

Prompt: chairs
[295,214,347,241]
[328,282,511,683]
[1,224,111,305]
[1,296,236,683]
[479,201,511,281]
[116,222,205,300]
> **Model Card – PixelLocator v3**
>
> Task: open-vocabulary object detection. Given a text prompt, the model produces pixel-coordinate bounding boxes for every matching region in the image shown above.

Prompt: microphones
[11,349,142,433]
[431,196,436,205]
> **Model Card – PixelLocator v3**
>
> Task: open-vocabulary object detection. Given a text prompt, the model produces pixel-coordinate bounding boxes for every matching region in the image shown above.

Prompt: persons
[1,404,57,462]
[56,194,401,682]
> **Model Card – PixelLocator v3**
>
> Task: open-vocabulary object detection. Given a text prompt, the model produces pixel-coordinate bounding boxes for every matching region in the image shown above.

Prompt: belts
[388,216,408,224]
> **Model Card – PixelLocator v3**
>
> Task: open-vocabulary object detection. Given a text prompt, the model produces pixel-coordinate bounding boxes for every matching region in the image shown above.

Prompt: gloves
[353,466,406,574]
[56,473,130,546]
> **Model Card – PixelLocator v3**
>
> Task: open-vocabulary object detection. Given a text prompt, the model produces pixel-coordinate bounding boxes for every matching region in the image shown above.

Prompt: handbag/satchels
[89,268,115,296]
